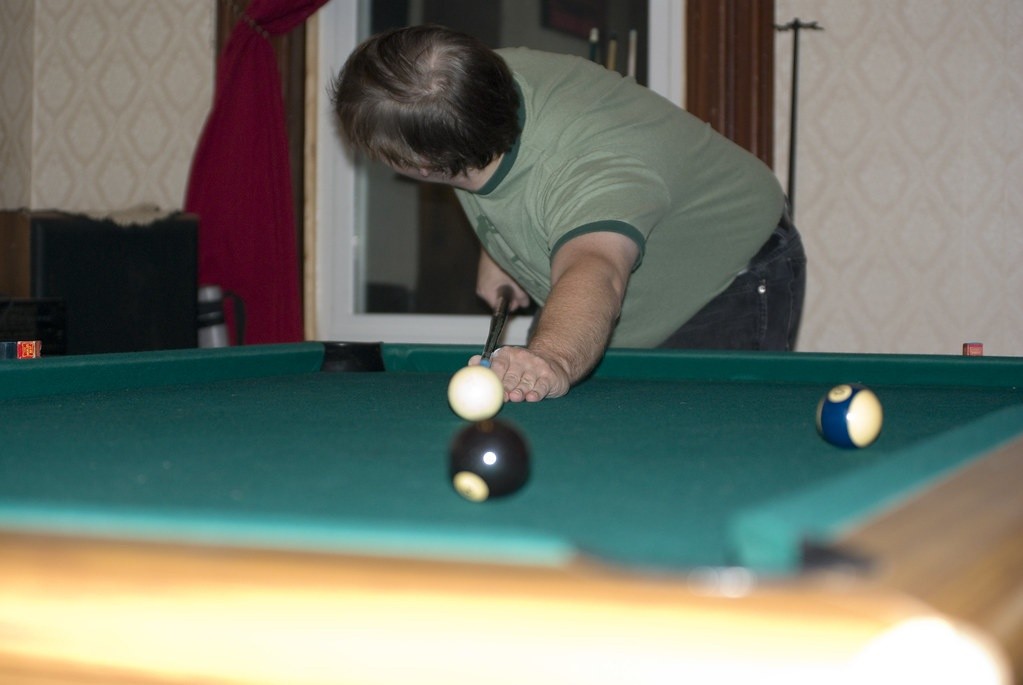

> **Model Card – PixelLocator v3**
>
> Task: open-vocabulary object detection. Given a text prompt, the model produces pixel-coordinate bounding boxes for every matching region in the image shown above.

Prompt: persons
[337,26,807,403]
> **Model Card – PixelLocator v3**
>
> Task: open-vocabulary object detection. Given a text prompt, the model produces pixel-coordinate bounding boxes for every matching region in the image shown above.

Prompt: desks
[0,341,1023,685]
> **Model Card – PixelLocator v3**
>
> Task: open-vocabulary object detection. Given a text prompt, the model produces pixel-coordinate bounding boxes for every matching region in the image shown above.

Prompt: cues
[479,283,516,368]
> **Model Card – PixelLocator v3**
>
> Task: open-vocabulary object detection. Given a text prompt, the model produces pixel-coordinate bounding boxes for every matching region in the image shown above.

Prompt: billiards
[447,366,504,422]
[448,419,531,505]
[813,382,884,449]
